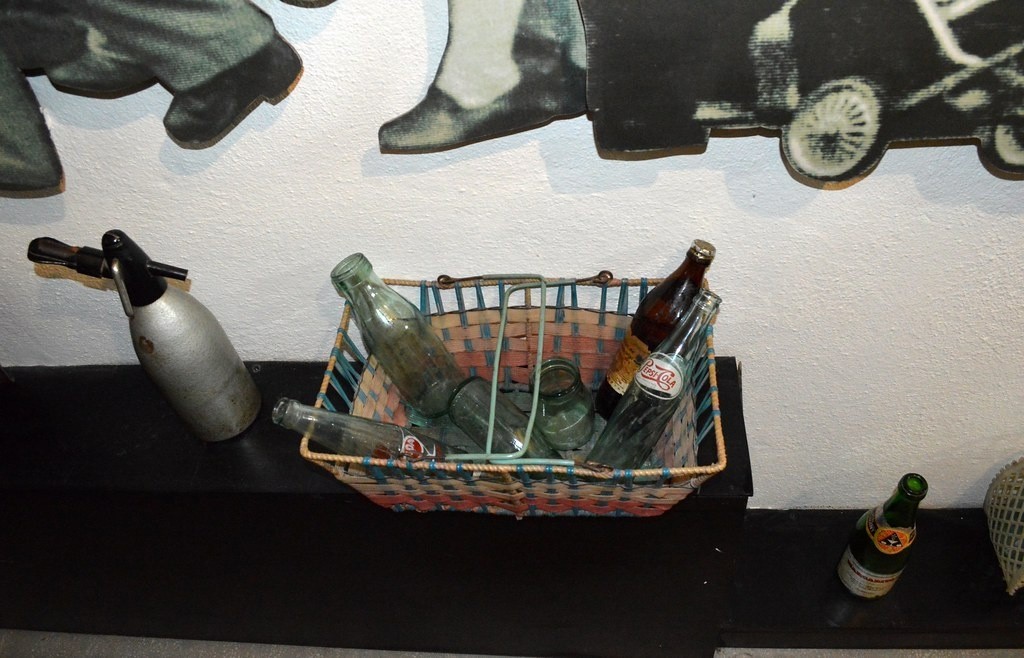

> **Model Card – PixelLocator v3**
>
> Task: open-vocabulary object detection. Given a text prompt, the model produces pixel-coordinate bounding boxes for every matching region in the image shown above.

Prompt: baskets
[301,272,725,519]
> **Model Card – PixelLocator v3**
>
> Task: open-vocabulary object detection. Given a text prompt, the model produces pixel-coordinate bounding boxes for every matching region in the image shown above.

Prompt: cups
[528,357,594,452]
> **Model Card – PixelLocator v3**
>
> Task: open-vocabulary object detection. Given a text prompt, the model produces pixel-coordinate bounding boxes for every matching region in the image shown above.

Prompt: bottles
[330,253,466,420]
[104,227,260,443]
[593,238,719,423]
[583,289,723,470]
[271,399,473,470]
[446,379,561,477]
[838,472,927,600]
